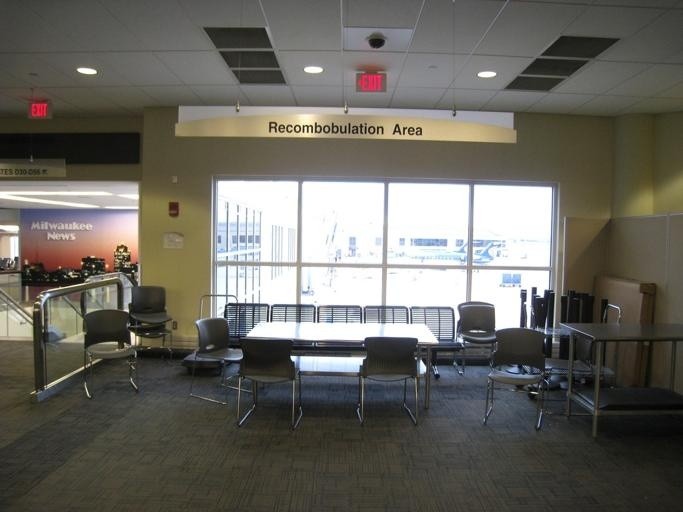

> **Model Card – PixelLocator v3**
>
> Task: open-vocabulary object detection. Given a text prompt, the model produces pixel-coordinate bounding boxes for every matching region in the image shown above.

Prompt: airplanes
[395,239,498,272]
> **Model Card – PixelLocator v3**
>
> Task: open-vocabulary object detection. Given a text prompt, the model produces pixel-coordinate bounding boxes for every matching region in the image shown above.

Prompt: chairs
[481,327,549,430]
[355,337,419,424]
[236,338,304,429]
[127,285,174,362]
[80,309,141,398]
[187,317,251,406]
[451,301,499,375]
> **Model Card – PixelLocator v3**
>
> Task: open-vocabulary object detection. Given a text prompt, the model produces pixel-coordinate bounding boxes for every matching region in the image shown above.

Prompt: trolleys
[512,302,622,400]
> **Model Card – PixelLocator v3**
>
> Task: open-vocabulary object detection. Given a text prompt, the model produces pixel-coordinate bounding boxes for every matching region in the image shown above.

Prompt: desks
[246,317,443,409]
[558,320,683,437]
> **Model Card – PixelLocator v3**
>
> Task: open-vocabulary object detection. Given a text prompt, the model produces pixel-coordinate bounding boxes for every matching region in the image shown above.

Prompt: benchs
[223,301,467,382]
